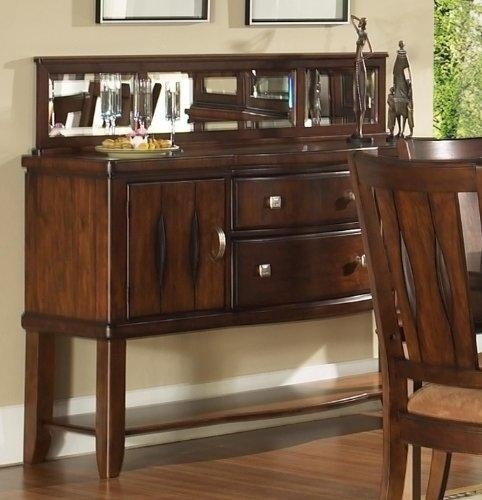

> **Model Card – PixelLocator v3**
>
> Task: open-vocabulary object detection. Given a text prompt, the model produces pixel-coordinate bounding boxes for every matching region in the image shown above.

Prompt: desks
[21,137,443,479]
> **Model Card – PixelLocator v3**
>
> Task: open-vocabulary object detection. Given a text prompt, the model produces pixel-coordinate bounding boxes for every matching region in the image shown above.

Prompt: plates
[94,144,179,159]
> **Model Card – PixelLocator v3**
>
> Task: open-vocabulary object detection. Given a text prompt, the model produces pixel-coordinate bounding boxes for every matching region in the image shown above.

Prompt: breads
[101,135,172,149]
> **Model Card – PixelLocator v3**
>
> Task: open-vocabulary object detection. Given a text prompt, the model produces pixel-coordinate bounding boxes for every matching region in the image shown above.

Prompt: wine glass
[99,71,184,153]
